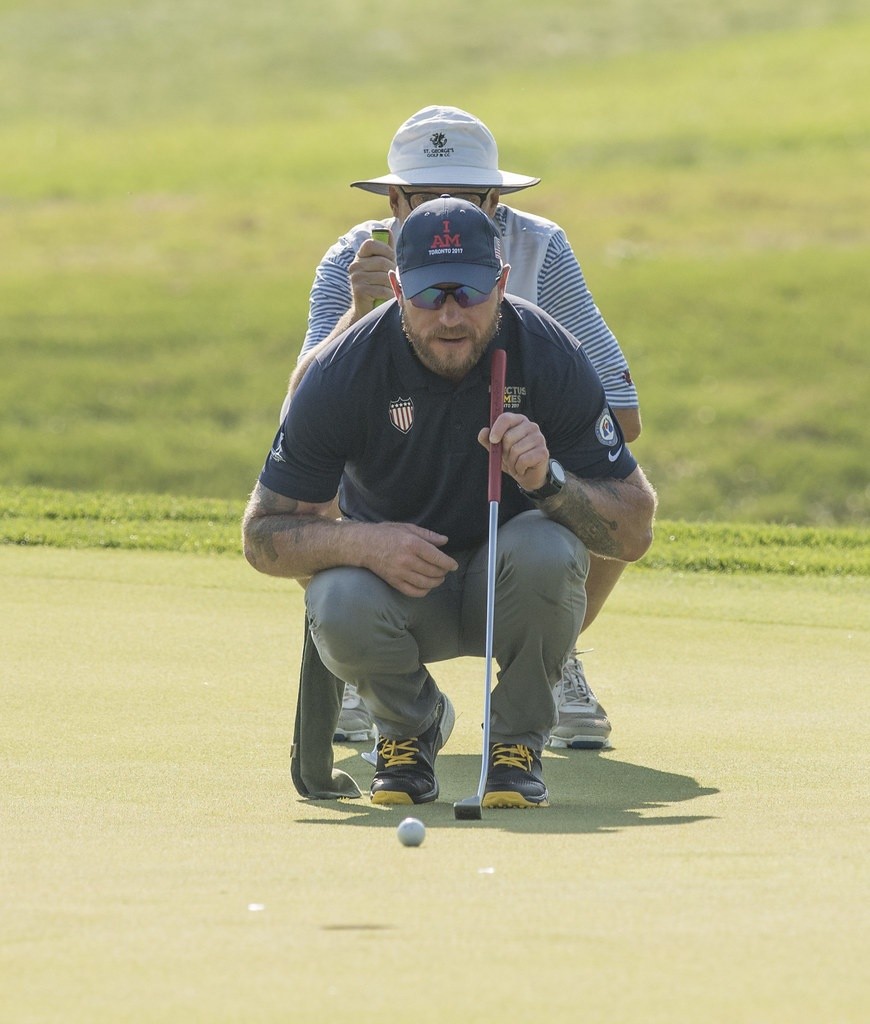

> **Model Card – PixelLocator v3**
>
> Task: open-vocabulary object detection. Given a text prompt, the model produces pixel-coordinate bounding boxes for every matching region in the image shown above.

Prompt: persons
[245,193,656,809]
[280,106,642,748]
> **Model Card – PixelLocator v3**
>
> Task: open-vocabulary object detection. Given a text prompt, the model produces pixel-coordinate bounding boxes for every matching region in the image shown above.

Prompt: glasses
[399,275,501,310]
[399,185,491,214]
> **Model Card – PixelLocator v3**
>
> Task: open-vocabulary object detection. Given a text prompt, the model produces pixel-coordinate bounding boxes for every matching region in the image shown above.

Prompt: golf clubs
[451,351,508,820]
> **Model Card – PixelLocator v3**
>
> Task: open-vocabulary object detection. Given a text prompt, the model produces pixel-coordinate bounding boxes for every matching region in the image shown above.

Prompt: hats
[395,194,503,299]
[350,105,541,196]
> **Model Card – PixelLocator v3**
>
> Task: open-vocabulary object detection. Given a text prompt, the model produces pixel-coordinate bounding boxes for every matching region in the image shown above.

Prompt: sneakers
[370,693,453,805]
[546,648,612,749]
[482,738,548,809]
[332,684,373,742]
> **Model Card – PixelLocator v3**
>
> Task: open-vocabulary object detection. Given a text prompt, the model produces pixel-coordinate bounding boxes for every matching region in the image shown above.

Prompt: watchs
[516,456,566,502]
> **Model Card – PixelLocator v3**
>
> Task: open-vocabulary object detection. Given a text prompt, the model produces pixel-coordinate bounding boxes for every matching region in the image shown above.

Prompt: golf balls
[396,820,433,850]
